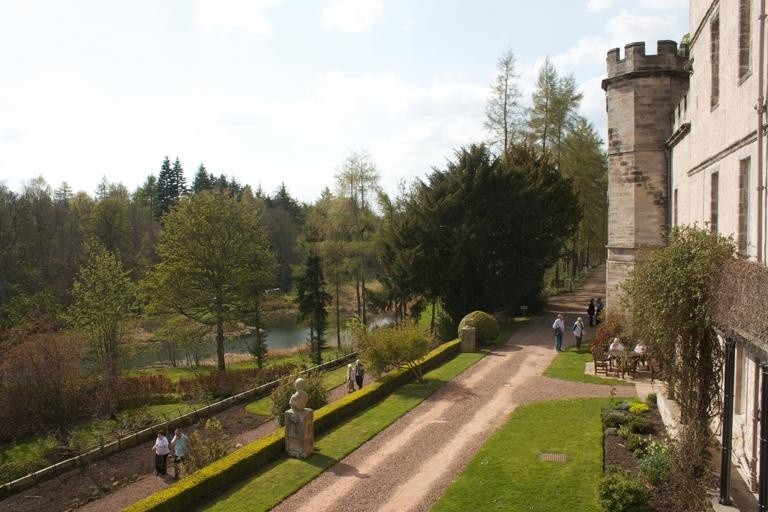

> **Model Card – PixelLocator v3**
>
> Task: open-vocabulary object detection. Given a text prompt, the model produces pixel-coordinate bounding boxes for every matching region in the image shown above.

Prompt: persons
[552,312,567,353]
[572,317,586,353]
[632,340,649,355]
[607,337,629,367]
[151,429,169,476]
[597,299,606,326]
[168,429,192,479]
[346,364,355,391]
[590,298,596,328]
[354,358,366,390]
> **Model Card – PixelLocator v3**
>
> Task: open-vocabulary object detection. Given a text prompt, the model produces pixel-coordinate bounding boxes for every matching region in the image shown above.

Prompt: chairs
[591,342,663,382]
